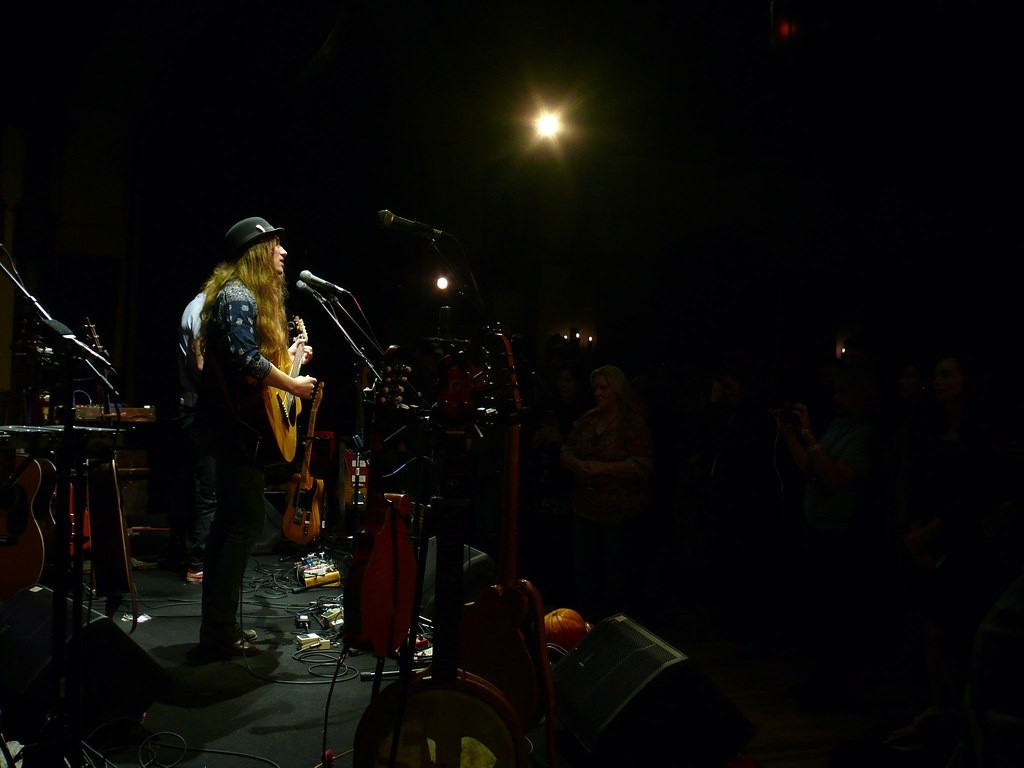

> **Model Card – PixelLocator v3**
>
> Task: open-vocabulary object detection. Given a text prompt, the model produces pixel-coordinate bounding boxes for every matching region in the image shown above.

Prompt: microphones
[347,530,371,547]
[297,280,330,304]
[379,209,455,240]
[300,269,353,296]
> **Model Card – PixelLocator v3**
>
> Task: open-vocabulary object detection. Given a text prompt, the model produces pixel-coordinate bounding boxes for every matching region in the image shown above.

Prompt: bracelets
[806,444,822,455]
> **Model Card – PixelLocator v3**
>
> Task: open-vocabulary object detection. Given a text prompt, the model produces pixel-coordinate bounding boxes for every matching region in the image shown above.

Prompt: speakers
[540,613,758,768]
[0,582,170,757]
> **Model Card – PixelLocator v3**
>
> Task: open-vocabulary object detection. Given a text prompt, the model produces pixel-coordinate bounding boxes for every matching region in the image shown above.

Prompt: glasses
[723,382,736,389]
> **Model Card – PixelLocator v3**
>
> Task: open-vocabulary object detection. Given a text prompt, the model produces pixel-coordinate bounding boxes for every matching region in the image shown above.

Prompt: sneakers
[187,568,203,582]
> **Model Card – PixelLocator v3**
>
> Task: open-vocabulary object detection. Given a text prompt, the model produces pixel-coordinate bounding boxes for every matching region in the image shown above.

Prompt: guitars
[466,322,541,738]
[82,313,137,597]
[281,378,326,545]
[0,432,59,601]
[240,312,306,468]
[349,333,527,767]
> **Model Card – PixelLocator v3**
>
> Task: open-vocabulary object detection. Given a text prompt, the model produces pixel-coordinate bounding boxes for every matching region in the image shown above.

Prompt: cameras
[779,401,804,427]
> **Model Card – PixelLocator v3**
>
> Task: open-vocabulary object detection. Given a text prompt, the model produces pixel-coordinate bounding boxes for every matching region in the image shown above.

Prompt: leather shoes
[200,630,262,657]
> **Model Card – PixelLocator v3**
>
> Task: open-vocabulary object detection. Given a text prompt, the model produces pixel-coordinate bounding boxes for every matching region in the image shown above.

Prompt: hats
[225,217,286,255]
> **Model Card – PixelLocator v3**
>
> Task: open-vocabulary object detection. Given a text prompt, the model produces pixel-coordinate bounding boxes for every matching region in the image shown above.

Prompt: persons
[413,335,995,721]
[177,217,318,658]
